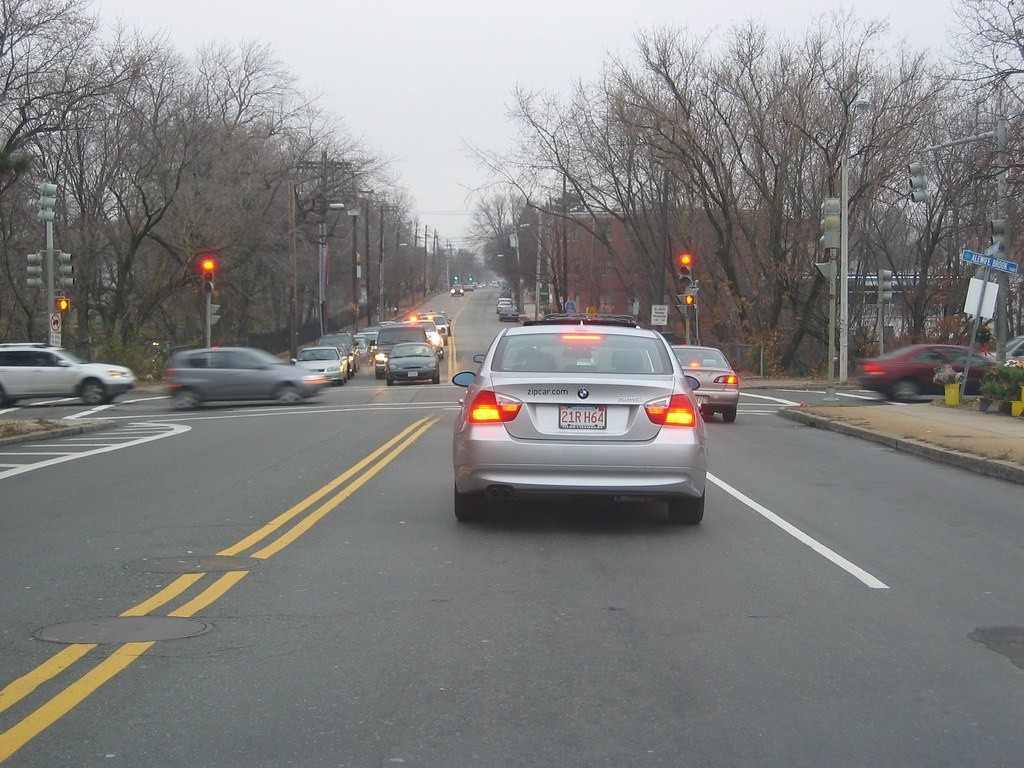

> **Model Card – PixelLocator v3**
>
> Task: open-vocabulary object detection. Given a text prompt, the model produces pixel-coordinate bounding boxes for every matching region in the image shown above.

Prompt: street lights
[380,231,408,322]
[316,201,345,337]
[839,98,871,388]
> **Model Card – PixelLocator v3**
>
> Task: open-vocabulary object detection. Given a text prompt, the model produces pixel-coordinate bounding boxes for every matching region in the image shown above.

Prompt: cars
[0,342,137,409]
[448,279,487,297]
[290,346,350,386]
[667,343,739,423]
[160,346,326,412]
[980,334,1024,365]
[490,280,518,322]
[290,309,454,388]
[854,343,1015,404]
[383,342,442,386]
[450,316,708,526]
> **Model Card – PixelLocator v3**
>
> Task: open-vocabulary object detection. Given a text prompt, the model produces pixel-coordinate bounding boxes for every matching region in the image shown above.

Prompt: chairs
[528,355,557,370]
[702,359,717,367]
[612,351,643,370]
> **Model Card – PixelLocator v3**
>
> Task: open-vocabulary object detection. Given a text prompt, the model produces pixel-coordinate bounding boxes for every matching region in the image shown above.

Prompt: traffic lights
[684,294,695,316]
[211,303,221,327]
[38,181,58,222]
[57,298,71,312]
[908,159,930,202]
[814,262,830,277]
[202,259,215,293]
[990,220,1006,260]
[58,251,75,288]
[878,269,893,302]
[26,249,44,289]
[679,254,693,287]
